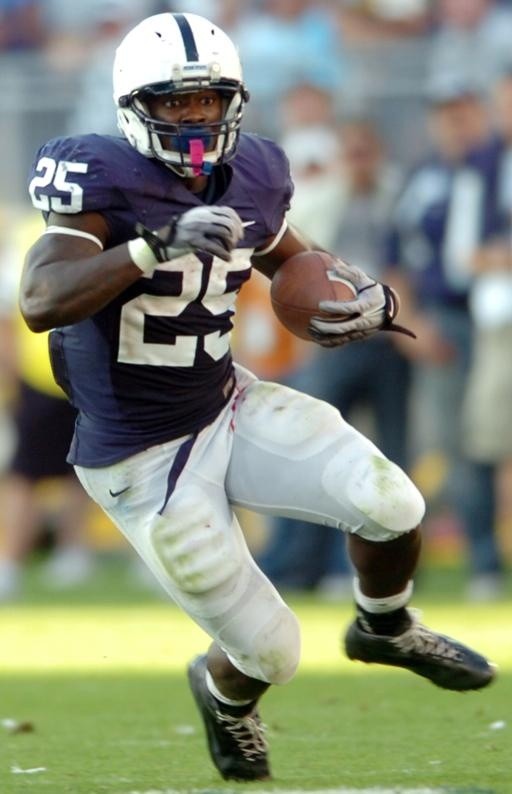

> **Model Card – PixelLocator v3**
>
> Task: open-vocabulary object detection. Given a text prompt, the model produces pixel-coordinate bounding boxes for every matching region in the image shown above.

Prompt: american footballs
[272,251,357,341]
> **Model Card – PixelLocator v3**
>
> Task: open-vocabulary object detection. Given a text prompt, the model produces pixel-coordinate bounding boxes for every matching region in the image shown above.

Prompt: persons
[19,11,493,780]
[0,0,512,603]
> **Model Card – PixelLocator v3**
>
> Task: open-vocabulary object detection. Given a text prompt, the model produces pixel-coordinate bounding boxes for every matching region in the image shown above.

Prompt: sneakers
[344,618,494,692]
[186,653,271,782]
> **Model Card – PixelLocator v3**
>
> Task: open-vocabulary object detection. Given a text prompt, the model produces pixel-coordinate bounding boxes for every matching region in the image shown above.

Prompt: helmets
[113,12,248,179]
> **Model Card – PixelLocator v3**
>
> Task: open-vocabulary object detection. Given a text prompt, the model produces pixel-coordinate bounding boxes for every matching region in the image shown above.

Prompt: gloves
[135,202,243,264]
[306,263,417,350]
